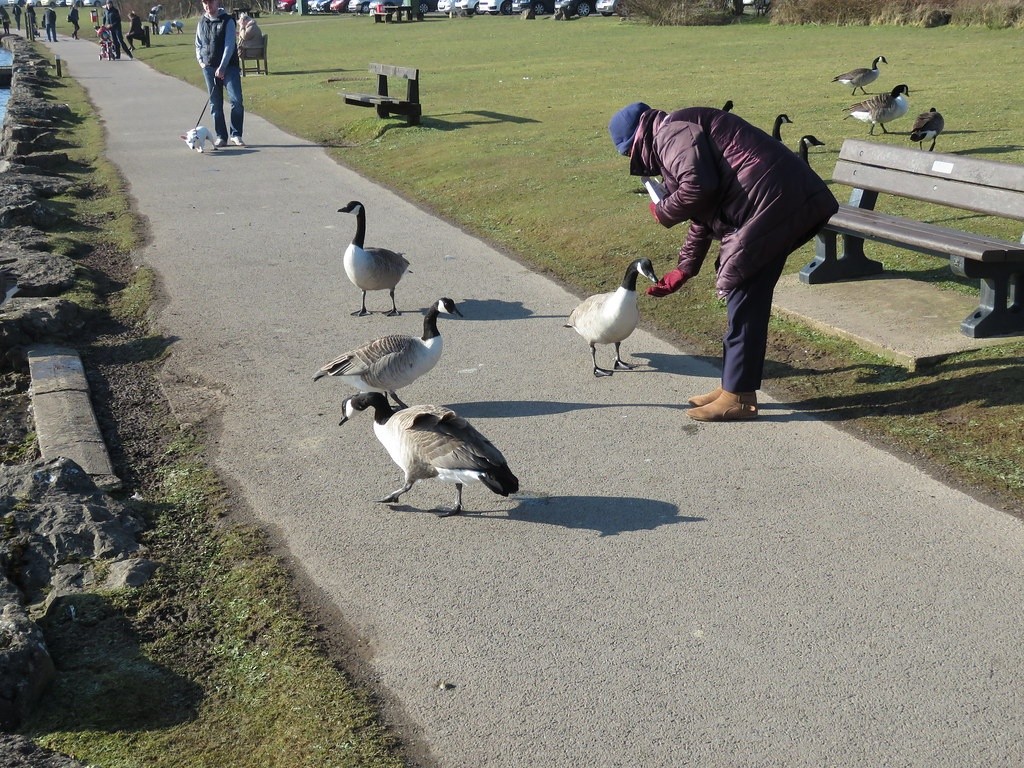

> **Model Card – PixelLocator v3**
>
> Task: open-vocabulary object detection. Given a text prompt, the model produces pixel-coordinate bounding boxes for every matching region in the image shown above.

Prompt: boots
[686,390,758,420]
[688,386,723,406]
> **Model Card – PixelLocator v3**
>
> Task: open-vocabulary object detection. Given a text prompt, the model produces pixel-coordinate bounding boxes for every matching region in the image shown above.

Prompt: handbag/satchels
[67,15,72,22]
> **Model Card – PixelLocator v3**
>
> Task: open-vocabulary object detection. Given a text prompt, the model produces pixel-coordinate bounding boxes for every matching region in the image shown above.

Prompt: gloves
[650,202,662,224]
[647,269,693,297]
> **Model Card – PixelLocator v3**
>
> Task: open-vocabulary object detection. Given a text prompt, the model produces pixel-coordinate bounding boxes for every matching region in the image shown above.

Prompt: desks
[233,7,251,20]
[383,6,412,22]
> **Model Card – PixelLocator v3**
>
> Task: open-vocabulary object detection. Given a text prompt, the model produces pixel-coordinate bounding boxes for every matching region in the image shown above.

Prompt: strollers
[32,23,40,38]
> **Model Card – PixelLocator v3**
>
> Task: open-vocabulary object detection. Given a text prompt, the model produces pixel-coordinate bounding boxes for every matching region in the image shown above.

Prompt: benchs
[799,139,1024,340]
[337,62,423,121]
[228,12,234,19]
[251,11,259,18]
[406,12,425,21]
[374,13,392,24]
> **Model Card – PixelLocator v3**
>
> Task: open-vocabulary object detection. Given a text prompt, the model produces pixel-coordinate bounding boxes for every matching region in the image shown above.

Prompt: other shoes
[49,39,51,42]
[231,137,245,146]
[128,47,131,50]
[72,34,74,38]
[75,37,79,39]
[131,48,135,51]
[214,139,227,147]
[54,39,58,42]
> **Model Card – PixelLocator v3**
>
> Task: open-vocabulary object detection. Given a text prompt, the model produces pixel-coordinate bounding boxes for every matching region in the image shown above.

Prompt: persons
[102,0,133,61]
[125,10,144,51]
[609,103,840,423]
[12,4,22,30]
[149,4,163,35]
[25,3,37,42]
[235,12,263,59]
[172,20,184,33]
[0,5,11,35]
[70,4,80,39]
[43,8,58,42]
[159,21,174,35]
[194,1,245,147]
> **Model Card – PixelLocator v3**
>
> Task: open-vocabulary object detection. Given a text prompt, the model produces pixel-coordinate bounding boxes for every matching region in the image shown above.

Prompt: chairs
[133,26,151,48]
[239,35,267,76]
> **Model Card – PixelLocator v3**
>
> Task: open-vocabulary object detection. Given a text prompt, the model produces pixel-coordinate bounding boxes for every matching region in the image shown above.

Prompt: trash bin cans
[90,11,97,22]
[403,0,420,21]
[297,0,308,14]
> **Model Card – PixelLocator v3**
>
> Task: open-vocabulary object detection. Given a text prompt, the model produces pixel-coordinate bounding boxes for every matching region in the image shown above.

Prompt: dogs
[180,126,219,153]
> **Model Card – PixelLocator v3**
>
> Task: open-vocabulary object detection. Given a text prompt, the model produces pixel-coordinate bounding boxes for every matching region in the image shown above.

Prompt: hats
[609,102,651,155]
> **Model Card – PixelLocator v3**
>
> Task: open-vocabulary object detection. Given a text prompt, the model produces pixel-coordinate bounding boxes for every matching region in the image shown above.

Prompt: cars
[277,0,333,14]
[347,0,375,14]
[83,0,107,8]
[437,0,461,16]
[402,0,439,14]
[511,0,555,15]
[554,0,597,17]
[8,0,84,7]
[595,0,618,17]
[455,0,485,15]
[330,0,350,13]
[725,0,771,16]
[478,0,513,16]
[369,0,404,13]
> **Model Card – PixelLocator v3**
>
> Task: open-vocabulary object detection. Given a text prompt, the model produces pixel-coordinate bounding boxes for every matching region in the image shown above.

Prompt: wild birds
[910,108,944,152]
[799,135,825,167]
[311,297,464,410]
[338,392,519,518]
[772,114,793,143]
[722,100,733,112]
[337,201,410,317]
[831,56,888,95]
[841,85,909,136]
[563,257,659,377]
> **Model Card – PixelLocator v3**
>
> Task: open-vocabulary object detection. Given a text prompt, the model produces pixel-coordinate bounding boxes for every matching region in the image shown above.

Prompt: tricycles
[94,25,115,61]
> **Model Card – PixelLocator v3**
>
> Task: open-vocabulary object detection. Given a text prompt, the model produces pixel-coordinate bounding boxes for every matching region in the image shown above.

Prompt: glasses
[624,141,633,157]
